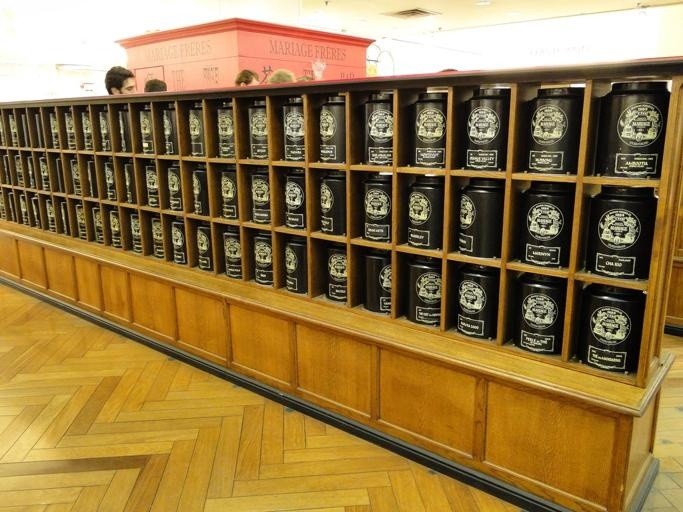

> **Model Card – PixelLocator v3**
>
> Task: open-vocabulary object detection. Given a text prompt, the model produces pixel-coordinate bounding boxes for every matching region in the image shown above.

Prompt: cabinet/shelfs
[0,56,682,512]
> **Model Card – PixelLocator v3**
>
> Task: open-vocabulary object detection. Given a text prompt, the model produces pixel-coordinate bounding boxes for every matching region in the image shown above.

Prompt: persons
[106,66,138,94]
[266,70,295,84]
[79,83,93,95]
[144,78,167,92]
[236,69,259,86]
